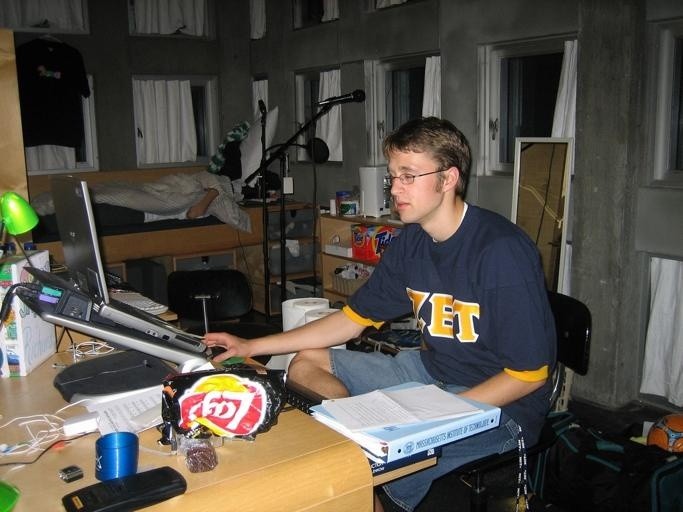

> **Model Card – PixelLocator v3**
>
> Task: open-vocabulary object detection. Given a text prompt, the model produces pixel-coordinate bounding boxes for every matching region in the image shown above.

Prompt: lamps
[0,191,39,245]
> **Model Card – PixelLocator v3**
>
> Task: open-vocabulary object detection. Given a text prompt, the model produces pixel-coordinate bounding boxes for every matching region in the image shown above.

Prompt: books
[310,382,501,474]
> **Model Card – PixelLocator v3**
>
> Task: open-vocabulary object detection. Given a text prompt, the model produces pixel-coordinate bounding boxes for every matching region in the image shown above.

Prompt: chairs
[168,269,260,339]
[462,291,592,512]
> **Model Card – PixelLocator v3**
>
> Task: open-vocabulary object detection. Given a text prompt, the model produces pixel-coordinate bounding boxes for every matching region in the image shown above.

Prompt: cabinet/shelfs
[319,213,402,305]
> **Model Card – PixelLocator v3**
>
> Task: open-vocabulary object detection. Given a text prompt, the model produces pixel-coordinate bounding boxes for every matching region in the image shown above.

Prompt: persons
[203,117,558,512]
[93,187,218,227]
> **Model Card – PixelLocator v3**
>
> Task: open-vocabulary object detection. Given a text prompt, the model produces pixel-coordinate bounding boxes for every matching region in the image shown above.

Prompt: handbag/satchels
[528,412,683,512]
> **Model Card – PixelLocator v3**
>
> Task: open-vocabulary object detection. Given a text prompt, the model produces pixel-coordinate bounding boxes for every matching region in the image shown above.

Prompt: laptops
[49,175,208,355]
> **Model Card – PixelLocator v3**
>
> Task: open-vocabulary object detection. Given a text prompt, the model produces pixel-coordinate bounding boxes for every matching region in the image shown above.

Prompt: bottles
[335,191,353,215]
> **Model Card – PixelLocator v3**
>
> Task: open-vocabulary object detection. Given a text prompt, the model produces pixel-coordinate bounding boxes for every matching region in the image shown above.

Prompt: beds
[27,165,320,325]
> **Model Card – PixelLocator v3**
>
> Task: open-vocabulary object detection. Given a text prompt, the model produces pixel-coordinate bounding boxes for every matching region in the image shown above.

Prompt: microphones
[320,85,369,107]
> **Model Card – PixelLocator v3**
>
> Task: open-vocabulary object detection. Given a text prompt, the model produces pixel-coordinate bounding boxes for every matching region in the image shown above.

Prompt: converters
[63,413,99,437]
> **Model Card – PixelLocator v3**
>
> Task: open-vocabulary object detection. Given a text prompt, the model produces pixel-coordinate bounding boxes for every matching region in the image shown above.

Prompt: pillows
[240,105,279,188]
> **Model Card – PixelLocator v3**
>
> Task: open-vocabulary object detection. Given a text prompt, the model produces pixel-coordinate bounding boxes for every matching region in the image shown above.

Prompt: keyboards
[283,386,317,414]
[109,290,169,318]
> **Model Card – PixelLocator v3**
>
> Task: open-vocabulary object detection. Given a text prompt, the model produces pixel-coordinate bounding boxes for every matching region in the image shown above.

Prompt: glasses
[386,170,444,186]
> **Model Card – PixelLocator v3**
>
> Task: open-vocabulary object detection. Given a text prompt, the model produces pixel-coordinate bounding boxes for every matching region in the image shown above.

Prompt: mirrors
[511,137,574,294]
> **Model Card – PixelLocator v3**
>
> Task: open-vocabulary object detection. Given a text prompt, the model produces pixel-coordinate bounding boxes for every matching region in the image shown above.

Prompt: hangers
[37,22,63,43]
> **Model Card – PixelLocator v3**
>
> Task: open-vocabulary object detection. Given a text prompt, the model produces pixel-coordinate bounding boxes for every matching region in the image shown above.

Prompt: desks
[0,309,437,512]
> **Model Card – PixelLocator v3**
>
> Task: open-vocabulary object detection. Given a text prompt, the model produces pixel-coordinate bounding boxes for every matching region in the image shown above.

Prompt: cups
[95,431,139,483]
